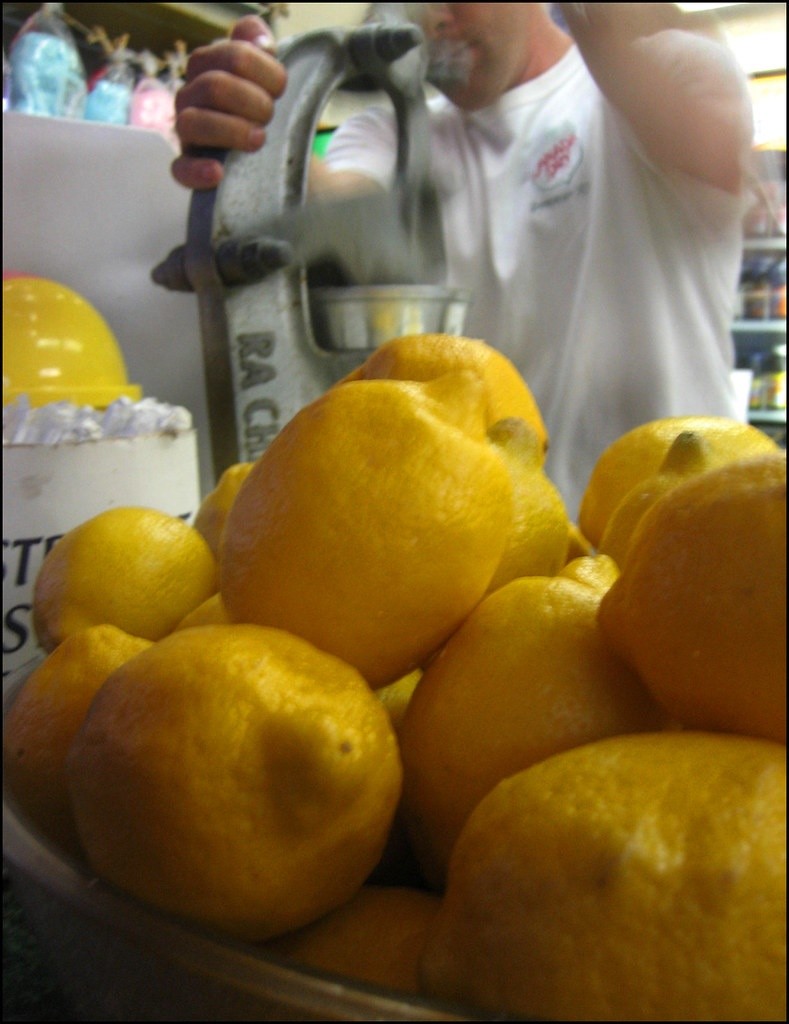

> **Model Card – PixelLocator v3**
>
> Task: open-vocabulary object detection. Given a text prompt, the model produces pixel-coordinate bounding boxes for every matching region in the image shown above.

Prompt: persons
[171,3,758,530]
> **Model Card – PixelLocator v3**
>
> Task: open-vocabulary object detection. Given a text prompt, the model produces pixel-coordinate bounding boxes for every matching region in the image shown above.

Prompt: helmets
[2,276,142,409]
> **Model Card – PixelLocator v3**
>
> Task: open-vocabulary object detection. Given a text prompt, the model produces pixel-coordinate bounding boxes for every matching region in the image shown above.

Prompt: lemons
[0,334,789,1021]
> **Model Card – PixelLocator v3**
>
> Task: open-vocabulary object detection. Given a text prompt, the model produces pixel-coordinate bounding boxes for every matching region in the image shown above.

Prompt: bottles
[733,334,787,410]
[736,247,786,320]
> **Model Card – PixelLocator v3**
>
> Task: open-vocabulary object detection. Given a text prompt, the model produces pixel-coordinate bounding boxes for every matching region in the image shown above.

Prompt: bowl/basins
[308,283,472,353]
